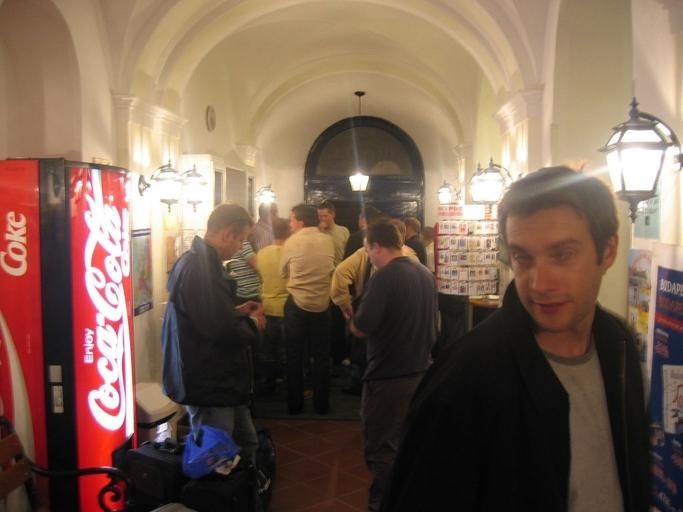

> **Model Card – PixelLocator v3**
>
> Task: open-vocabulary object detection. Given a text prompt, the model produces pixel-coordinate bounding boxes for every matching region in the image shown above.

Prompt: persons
[222,199,439,480]
[158,203,268,472]
[368,165,655,510]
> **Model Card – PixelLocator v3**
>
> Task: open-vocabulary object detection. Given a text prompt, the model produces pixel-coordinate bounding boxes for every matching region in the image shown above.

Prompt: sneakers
[304,390,312,399]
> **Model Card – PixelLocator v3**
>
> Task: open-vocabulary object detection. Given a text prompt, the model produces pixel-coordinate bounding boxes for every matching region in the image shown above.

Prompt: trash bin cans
[135,381,184,446]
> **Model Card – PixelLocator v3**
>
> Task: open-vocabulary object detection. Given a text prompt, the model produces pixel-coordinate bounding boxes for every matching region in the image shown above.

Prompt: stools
[466,294,501,332]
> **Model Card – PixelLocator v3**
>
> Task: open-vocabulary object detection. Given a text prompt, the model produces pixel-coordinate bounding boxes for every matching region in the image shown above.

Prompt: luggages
[124,438,259,511]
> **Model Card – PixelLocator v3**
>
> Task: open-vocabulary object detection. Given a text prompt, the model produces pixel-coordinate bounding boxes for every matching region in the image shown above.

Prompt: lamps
[597,96,683,223]
[438,180,461,206]
[137,159,208,214]
[469,157,512,206]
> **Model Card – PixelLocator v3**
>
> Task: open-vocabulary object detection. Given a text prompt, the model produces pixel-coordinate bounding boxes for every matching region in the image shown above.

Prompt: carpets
[253,361,360,420]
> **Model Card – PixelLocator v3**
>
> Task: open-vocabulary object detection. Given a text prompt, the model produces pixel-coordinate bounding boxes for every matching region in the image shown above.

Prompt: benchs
[0,417,134,512]
